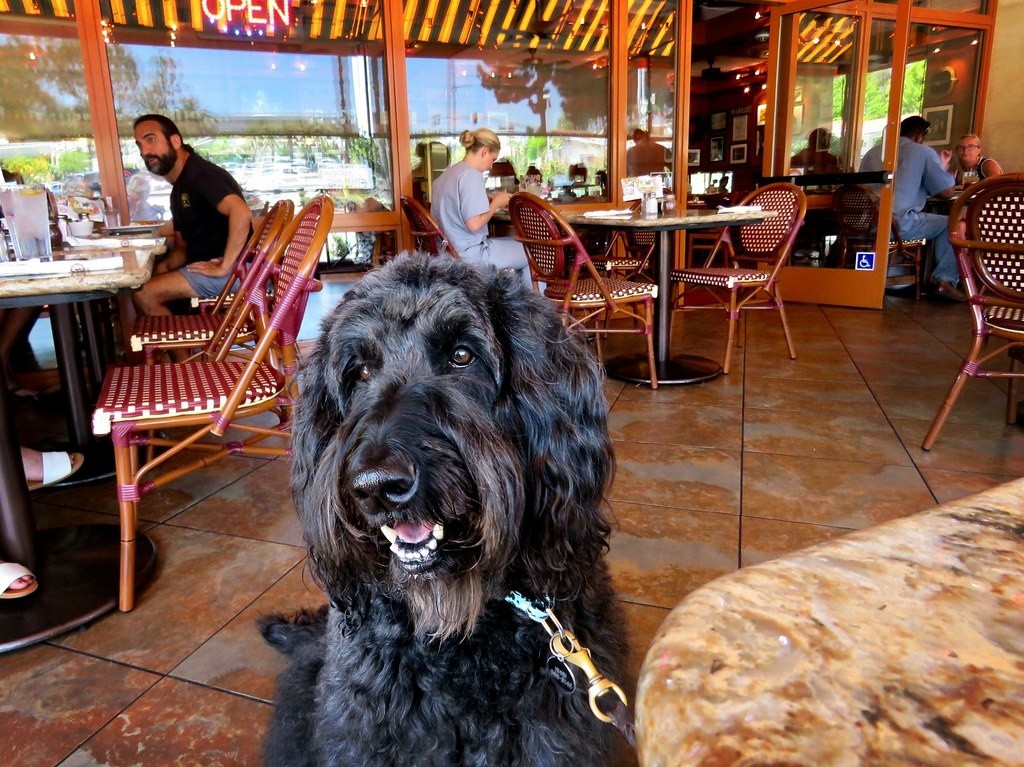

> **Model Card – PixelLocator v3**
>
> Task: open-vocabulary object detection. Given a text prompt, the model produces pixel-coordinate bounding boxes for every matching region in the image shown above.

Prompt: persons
[131,114,256,363]
[0,306,84,598]
[711,176,729,194]
[627,129,673,181]
[858,116,966,301]
[940,134,1003,194]
[431,128,546,297]
[792,128,837,173]
[125,176,165,222]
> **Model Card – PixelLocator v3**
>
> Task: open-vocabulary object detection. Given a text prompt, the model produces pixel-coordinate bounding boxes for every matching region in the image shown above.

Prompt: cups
[595,175,601,185]
[0,185,54,262]
[99,196,121,227]
[548,178,554,190]
[962,171,979,191]
[593,191,600,197]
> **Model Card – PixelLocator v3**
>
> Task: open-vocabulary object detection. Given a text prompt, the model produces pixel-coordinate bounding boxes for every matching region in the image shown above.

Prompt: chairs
[918,171,1024,452]
[90,194,335,613]
[398,182,806,392]
[829,182,925,300]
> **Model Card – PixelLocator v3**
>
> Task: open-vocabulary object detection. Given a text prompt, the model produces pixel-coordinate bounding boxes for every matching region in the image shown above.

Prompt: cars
[47,162,372,221]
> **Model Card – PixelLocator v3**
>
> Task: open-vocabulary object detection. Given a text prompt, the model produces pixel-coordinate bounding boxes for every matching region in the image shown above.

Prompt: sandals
[0,563,38,598]
[27,451,84,493]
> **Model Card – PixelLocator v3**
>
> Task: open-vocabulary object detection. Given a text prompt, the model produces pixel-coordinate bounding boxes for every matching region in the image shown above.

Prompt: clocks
[921,67,957,100]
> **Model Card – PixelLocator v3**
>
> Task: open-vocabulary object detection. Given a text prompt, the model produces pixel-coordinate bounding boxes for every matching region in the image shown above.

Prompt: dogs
[254,246,628,767]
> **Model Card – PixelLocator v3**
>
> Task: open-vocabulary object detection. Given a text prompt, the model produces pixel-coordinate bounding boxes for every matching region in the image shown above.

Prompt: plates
[100,223,165,235]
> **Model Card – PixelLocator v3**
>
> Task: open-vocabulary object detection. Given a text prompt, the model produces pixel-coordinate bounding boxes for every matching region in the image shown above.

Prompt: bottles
[0,220,12,262]
[526,166,542,187]
[545,193,553,203]
[56,215,72,242]
[569,163,586,182]
[641,192,658,213]
[526,175,536,195]
[78,213,90,222]
[661,195,675,212]
[49,220,62,246]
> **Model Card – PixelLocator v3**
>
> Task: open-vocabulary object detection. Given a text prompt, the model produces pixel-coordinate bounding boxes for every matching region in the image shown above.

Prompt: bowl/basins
[68,222,94,238]
[558,192,576,203]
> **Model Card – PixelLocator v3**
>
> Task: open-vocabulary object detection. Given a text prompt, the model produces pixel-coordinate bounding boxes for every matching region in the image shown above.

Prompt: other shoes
[936,283,966,303]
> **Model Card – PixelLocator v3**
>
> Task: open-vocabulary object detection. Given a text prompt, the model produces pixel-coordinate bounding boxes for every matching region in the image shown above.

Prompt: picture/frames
[816,118,845,152]
[663,82,804,167]
[649,171,673,193]
[918,103,954,147]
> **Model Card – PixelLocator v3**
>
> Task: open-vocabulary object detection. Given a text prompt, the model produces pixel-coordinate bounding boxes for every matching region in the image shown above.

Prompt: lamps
[701,58,720,79]
[755,11,769,20]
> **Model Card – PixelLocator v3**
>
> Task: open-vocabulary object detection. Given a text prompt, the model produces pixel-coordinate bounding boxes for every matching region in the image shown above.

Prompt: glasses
[957,144,980,151]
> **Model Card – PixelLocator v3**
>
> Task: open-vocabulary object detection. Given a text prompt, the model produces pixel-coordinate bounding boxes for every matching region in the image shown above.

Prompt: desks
[0,232,174,489]
[555,211,780,387]
[0,253,159,652]
[488,213,633,339]
